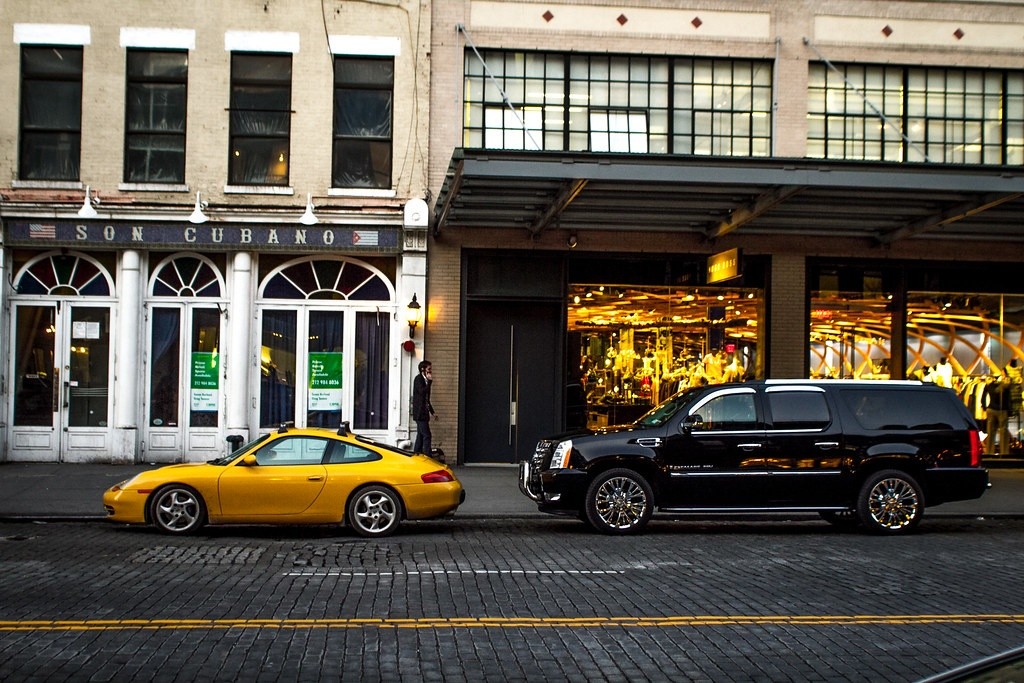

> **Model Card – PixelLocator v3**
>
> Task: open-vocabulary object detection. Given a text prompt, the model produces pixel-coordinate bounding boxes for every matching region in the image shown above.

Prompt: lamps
[77,185,100,218]
[189,191,209,224]
[616,288,623,299]
[298,192,319,225]
[407,293,421,338]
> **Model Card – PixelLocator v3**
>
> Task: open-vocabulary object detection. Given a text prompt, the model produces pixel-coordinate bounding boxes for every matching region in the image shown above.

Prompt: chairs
[331,444,346,462]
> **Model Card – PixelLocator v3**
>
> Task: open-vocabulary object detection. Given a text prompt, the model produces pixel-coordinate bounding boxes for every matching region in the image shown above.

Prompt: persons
[1006,357,1023,379]
[620,348,637,367]
[741,372,755,383]
[981,380,1013,454]
[922,356,954,389]
[702,349,722,377]
[412,359,438,456]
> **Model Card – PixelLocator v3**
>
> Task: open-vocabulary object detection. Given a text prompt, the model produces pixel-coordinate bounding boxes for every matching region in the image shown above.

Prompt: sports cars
[103,421,466,538]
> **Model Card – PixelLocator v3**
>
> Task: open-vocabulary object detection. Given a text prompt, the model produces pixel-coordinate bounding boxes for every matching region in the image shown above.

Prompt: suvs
[518,379,990,535]
